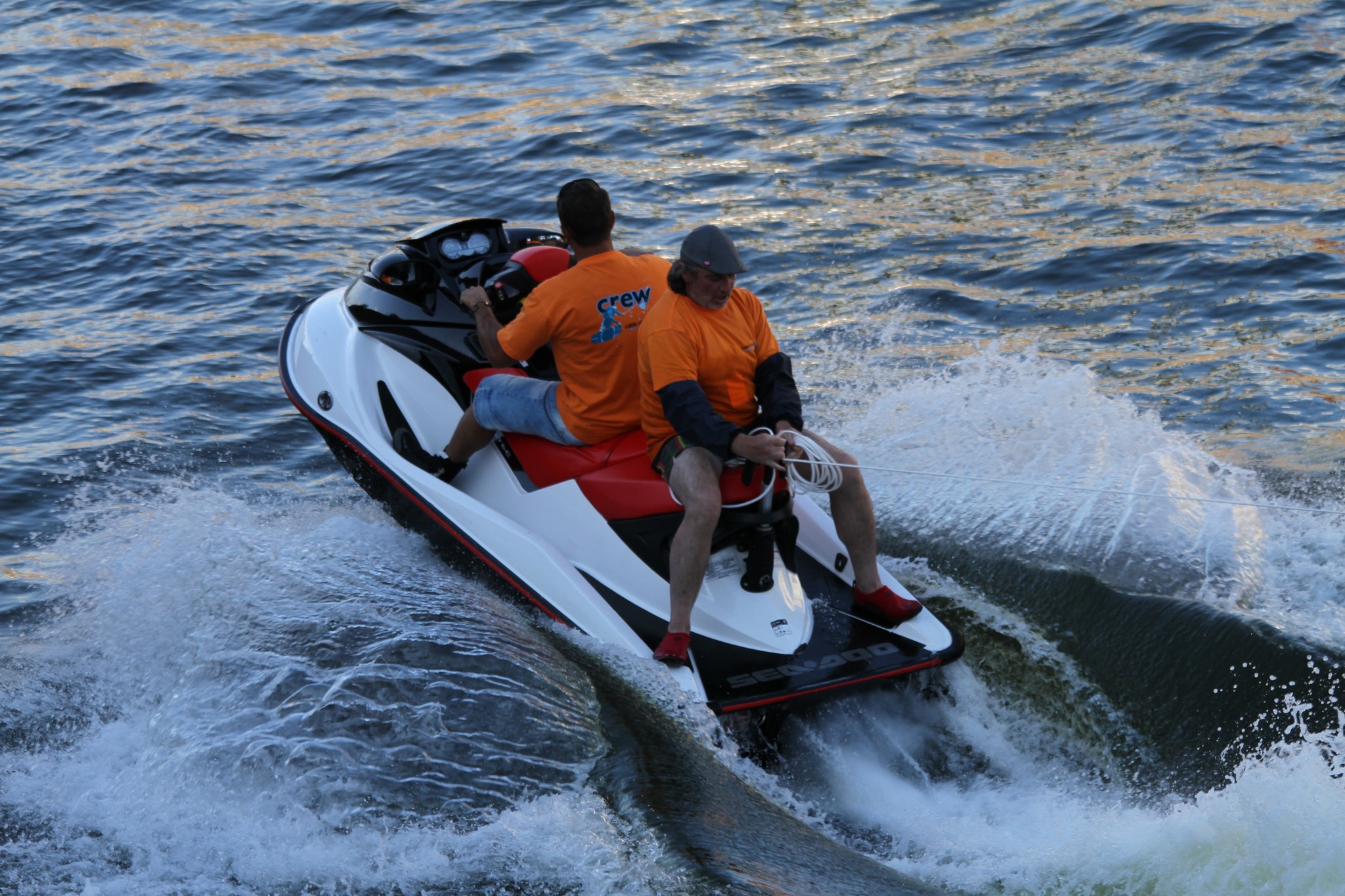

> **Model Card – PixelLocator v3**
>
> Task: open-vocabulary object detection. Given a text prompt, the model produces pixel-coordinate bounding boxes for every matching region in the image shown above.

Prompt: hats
[681,225,748,275]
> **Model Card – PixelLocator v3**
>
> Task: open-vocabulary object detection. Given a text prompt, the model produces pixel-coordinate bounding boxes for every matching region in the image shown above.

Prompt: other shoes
[653,631,692,664]
[853,583,923,621]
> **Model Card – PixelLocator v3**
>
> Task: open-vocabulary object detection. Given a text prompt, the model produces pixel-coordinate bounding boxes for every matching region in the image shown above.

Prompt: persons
[638,224,924,671]
[392,177,673,483]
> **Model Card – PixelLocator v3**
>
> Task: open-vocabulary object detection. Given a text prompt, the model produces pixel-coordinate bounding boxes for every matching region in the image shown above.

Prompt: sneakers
[393,428,468,484]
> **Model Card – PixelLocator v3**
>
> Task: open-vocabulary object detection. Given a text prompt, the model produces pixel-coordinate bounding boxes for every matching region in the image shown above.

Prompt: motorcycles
[277,215,967,722]
